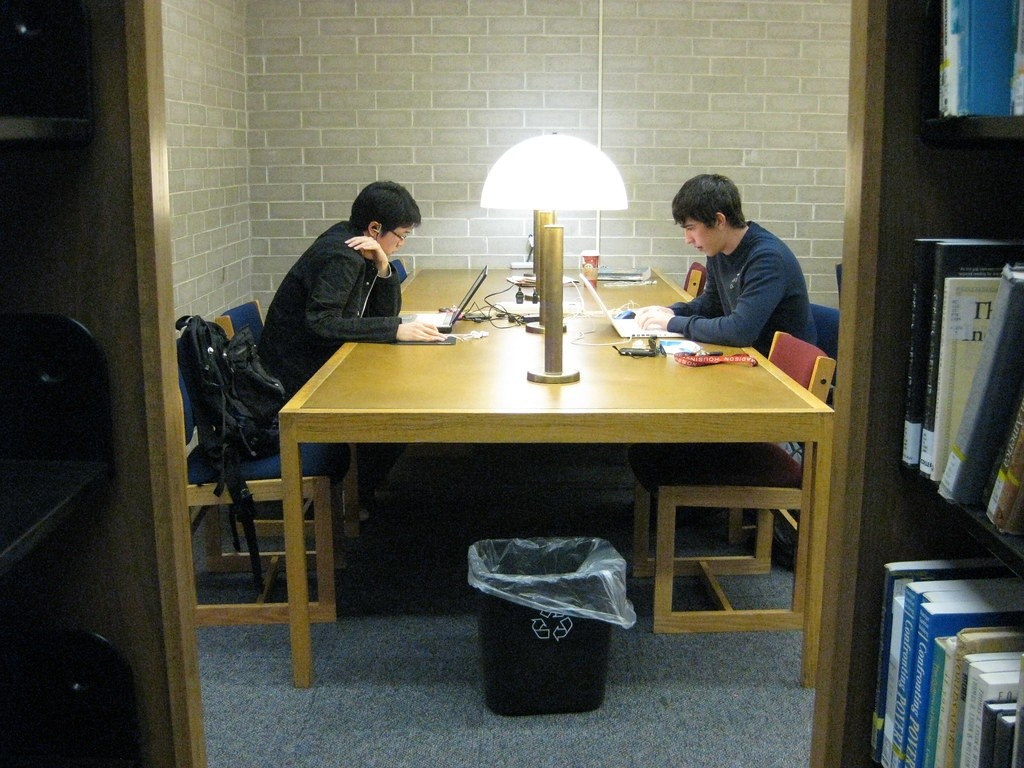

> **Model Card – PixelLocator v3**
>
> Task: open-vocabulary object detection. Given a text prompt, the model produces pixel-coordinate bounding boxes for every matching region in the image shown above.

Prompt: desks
[279,270,832,689]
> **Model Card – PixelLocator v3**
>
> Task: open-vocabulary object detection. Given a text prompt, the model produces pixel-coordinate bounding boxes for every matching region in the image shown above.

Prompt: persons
[258,181,448,490]
[611,174,818,527]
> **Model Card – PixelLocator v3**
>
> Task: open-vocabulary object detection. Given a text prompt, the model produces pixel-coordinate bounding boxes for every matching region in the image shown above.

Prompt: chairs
[174,341,348,624]
[683,260,706,296]
[631,329,835,634]
[214,299,360,539]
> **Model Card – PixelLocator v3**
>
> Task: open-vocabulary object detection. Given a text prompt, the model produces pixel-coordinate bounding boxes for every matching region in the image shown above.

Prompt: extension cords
[495,302,582,314]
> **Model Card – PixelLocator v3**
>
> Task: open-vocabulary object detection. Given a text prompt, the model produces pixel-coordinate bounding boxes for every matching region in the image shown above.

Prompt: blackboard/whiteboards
[466,536,628,718]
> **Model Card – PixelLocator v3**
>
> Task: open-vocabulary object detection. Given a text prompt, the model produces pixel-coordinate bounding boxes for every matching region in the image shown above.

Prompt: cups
[581,249,599,288]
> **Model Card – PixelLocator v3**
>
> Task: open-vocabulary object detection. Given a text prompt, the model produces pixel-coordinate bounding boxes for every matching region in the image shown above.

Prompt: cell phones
[618,347,656,357]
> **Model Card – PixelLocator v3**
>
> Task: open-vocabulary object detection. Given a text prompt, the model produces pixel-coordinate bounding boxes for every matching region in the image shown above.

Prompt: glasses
[389,228,404,243]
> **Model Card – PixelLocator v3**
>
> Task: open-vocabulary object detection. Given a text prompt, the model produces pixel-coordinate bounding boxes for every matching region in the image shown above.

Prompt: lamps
[480,131,631,319]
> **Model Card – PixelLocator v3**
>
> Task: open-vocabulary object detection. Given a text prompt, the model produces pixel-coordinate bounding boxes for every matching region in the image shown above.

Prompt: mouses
[615,310,636,319]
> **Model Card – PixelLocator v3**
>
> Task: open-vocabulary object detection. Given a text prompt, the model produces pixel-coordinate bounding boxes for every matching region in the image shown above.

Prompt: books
[868,1,1024,768]
[598,267,652,282]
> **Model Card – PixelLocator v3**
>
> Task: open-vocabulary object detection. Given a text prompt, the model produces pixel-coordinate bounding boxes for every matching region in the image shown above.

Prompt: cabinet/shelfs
[809,0,1024,768]
[0,113,106,582]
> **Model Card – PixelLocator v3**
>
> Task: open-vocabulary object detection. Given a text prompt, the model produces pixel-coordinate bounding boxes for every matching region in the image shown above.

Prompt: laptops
[399,265,489,334]
[579,273,685,338]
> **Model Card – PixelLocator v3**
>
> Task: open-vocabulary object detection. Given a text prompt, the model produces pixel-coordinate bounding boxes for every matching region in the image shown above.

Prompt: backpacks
[176,315,291,465]
